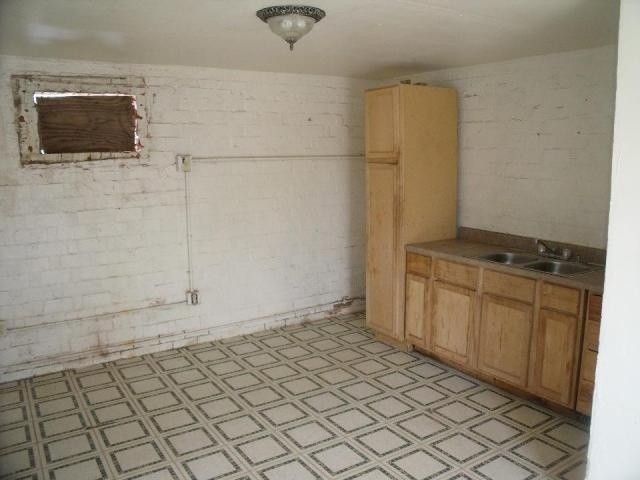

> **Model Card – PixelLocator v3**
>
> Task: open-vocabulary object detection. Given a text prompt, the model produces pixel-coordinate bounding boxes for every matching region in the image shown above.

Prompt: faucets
[535,239,558,254]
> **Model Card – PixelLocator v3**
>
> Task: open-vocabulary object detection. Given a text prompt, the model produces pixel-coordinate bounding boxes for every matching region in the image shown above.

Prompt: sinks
[524,261,598,278]
[480,252,538,264]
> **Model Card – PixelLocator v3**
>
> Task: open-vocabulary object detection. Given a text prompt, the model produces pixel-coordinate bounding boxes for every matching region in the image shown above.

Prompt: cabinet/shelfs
[364,85,461,348]
[400,250,602,420]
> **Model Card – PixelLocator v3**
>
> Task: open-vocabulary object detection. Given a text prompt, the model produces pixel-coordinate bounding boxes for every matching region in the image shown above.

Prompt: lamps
[256,7,326,53]
